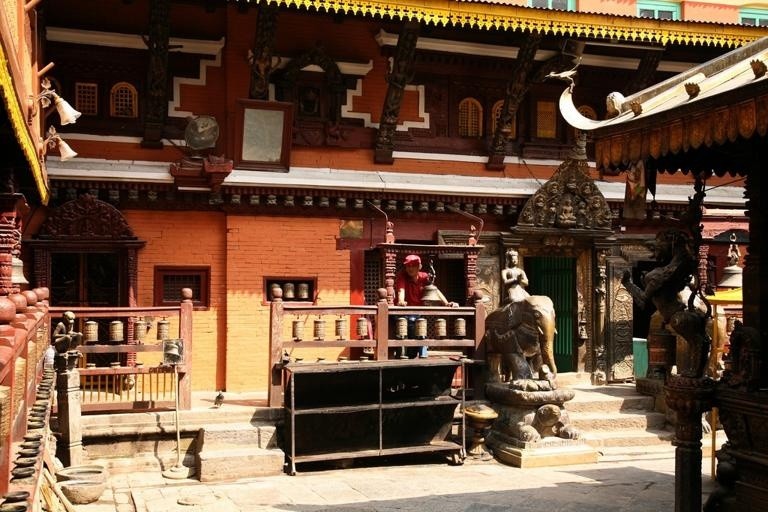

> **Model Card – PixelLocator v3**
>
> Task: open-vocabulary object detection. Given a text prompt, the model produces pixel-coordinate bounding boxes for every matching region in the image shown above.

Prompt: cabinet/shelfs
[281,353,467,476]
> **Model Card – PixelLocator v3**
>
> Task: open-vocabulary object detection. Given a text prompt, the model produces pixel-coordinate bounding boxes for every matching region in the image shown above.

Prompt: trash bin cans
[632,338,649,381]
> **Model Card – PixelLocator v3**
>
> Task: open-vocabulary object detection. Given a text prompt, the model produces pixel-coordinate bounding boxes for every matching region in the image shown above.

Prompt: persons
[558,194,577,220]
[500,250,530,303]
[395,254,429,307]
[621,214,710,379]
[53,311,86,357]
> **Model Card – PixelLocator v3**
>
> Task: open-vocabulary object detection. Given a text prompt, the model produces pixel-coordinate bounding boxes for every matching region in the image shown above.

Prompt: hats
[403,255,421,264]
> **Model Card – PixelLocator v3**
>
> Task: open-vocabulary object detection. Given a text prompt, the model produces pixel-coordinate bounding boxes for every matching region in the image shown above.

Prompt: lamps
[39,125,78,162]
[27,77,83,126]
